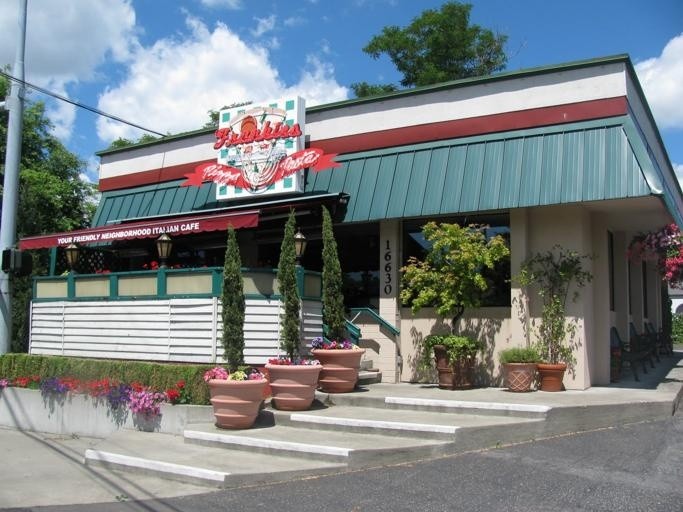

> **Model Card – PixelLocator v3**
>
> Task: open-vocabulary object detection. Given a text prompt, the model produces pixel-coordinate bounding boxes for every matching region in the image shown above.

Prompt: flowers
[270,356,321,368]
[206,362,264,383]
[626,223,683,283]
[314,335,360,352]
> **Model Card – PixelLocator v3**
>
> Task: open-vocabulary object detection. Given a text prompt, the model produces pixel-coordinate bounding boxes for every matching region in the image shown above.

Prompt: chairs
[609,320,675,382]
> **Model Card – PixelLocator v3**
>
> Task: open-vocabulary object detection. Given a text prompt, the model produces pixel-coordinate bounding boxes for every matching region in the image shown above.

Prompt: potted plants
[395,221,598,395]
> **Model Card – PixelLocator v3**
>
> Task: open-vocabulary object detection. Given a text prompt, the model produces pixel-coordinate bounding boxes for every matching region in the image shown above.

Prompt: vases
[311,347,366,393]
[210,379,267,429]
[267,367,323,410]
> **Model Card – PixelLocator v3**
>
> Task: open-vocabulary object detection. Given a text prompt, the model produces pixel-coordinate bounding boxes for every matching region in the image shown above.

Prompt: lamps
[155,233,173,264]
[293,225,309,259]
[65,236,79,267]
